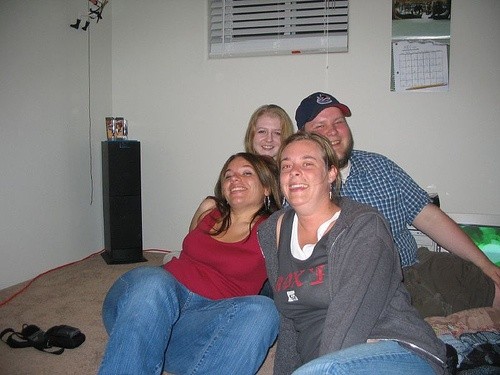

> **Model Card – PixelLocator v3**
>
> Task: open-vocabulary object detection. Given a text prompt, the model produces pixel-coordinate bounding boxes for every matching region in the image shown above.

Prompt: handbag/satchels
[0,323,86,355]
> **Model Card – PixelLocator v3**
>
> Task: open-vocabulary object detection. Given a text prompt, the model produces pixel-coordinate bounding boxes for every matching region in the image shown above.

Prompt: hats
[295,92,351,130]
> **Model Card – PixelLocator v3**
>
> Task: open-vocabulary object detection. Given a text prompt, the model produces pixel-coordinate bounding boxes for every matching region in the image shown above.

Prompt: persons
[244,104,295,159]
[280,92,500,320]
[256,130,451,375]
[97,152,281,375]
